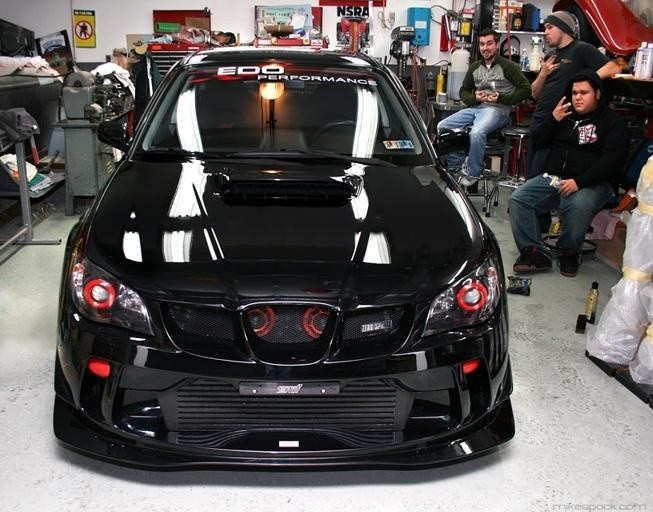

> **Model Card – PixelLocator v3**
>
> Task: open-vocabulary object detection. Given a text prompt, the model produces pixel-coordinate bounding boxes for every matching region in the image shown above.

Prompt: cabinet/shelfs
[0,58,73,199]
[477,0,546,99]
[603,75,652,190]
[0,128,62,267]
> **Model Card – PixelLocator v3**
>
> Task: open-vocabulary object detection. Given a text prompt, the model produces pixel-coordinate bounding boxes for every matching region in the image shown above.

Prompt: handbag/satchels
[433,129,478,195]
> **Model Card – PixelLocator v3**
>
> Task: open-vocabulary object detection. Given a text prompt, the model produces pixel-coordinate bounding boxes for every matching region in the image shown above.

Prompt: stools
[481,126,530,219]
[456,138,505,217]
[540,192,617,267]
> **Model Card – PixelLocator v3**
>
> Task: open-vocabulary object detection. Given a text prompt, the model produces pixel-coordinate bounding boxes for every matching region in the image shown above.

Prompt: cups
[439,94,448,103]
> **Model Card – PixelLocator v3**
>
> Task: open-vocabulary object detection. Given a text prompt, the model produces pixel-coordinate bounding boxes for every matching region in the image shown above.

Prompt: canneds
[438,93,447,104]
[633,49,653,79]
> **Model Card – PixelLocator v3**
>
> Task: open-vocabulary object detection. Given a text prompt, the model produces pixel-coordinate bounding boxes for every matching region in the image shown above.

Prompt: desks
[428,96,467,112]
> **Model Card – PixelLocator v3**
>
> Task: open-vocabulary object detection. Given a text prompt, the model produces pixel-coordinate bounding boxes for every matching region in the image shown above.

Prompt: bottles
[519,48,529,71]
[585,281,599,324]
[542,173,563,191]
[640,44,653,79]
[634,42,647,78]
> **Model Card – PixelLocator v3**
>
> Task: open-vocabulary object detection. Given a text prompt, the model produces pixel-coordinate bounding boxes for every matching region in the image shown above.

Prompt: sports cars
[53,45,514,475]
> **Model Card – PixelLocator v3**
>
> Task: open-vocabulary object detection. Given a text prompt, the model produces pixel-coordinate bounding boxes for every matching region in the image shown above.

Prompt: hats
[543,10,576,40]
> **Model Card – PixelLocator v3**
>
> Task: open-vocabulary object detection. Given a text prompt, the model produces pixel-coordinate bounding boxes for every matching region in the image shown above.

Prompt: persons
[525,10,622,233]
[509,69,629,277]
[436,27,532,195]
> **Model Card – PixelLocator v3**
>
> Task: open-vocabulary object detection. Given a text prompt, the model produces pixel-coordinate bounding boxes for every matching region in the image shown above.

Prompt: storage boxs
[595,222,626,275]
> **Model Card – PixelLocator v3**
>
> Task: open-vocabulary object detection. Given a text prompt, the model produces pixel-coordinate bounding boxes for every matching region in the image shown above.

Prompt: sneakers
[461,176,484,187]
[558,249,580,277]
[513,251,553,273]
